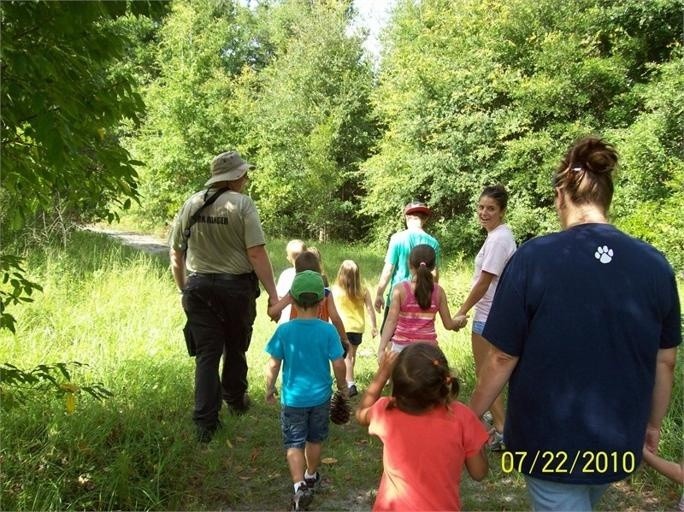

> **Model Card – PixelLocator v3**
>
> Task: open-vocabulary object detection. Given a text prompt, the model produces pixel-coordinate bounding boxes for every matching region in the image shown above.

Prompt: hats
[203,151,258,186]
[288,272,330,304]
[403,199,433,219]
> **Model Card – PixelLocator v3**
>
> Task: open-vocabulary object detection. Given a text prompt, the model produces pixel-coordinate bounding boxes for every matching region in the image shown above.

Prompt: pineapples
[331,407,350,424]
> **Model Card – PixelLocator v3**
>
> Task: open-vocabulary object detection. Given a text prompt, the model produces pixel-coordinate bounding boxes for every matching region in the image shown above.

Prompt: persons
[329,259,379,396]
[277,238,308,327]
[377,244,470,358]
[306,247,325,276]
[465,132,682,512]
[168,149,281,445]
[452,183,519,452]
[263,269,351,512]
[267,250,351,360]
[373,200,442,386]
[352,341,490,512]
[640,439,683,488]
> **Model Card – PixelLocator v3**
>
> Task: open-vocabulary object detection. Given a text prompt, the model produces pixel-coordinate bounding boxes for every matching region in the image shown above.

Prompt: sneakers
[287,470,322,511]
[227,393,257,421]
[191,418,227,446]
[488,430,510,452]
[347,384,359,400]
